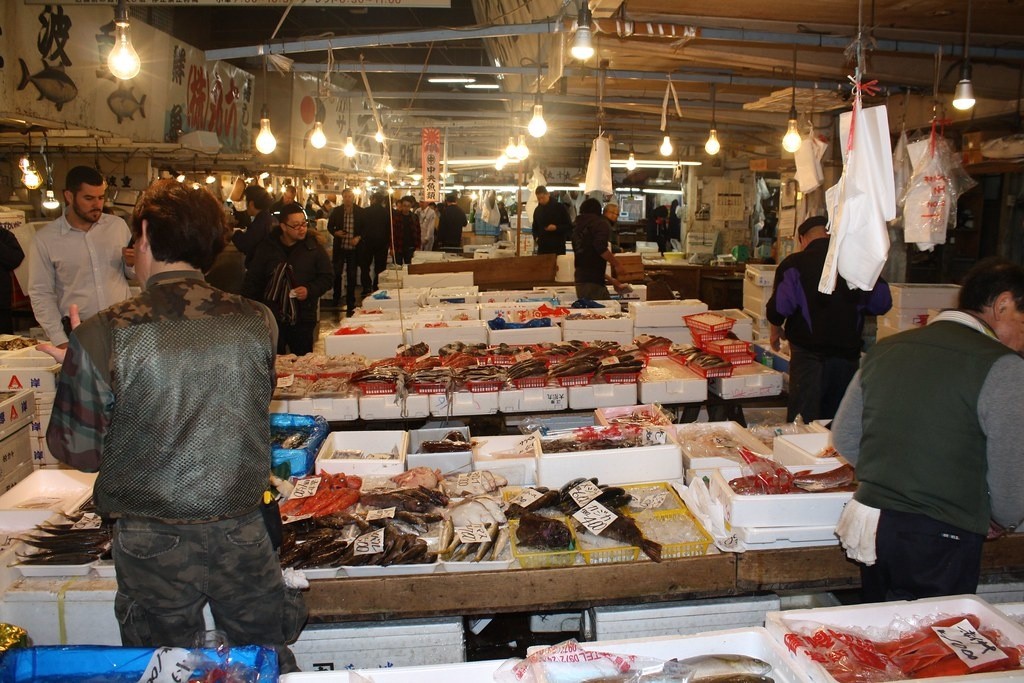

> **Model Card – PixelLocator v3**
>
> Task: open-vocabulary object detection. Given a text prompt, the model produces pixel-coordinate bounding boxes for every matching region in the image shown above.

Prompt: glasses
[284,221,308,230]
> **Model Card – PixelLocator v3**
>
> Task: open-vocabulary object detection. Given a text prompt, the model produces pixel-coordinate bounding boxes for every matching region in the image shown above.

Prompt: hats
[798,215,827,244]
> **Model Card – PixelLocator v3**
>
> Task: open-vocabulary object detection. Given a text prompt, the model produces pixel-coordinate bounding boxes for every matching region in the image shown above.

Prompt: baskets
[275,312,756,400]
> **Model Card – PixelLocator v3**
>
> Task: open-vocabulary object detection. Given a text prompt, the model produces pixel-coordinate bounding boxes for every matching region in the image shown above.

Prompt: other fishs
[271,467,663,572]
[669,343,734,371]
[578,652,775,683]
[5,510,112,570]
[728,462,855,496]
[351,340,645,425]
[820,614,1024,683]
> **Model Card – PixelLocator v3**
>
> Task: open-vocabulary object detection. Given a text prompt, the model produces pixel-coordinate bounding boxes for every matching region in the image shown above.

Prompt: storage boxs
[0,241,1024,683]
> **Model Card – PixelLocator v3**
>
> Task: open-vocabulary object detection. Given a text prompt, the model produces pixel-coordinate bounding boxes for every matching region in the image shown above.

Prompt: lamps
[952,0,976,109]
[42,140,60,211]
[570,0,594,58]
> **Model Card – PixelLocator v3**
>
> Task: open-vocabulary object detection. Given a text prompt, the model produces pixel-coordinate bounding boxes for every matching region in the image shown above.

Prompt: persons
[646,204,674,256]
[46,179,305,675]
[571,198,626,300]
[0,224,25,335]
[224,184,468,357]
[29,166,136,349]
[532,186,571,255]
[469,202,477,223]
[831,255,1024,602]
[766,215,893,430]
[497,201,511,227]
